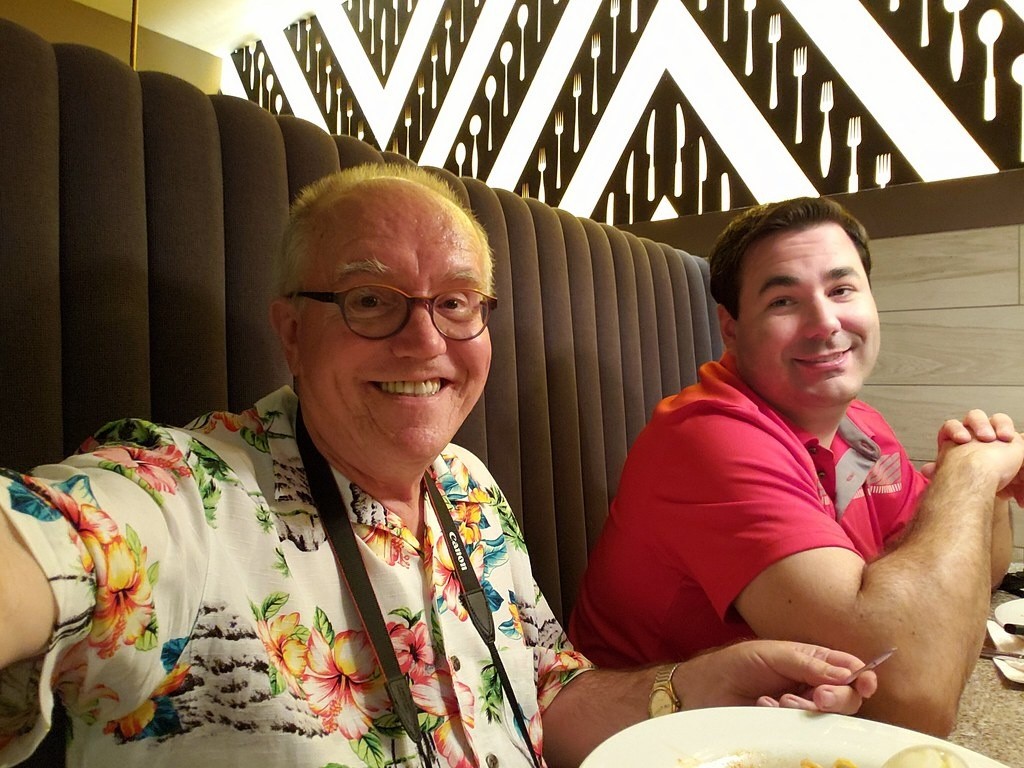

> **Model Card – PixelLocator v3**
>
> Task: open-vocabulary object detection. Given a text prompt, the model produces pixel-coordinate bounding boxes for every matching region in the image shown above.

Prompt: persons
[568,198,1024,739]
[0,162,879,768]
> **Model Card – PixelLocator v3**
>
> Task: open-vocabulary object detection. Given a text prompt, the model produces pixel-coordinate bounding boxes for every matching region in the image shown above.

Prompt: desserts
[882,745,971,768]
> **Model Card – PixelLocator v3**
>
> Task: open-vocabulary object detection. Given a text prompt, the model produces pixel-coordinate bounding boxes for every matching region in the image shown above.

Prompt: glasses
[284,284,498,341]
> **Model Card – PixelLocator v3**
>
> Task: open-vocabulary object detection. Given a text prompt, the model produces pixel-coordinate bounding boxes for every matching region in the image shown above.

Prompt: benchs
[0,13,739,629]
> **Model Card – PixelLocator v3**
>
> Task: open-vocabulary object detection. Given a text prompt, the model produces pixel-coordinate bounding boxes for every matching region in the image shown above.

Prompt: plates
[992,654,1024,683]
[995,598,1024,640]
[578,707,1010,768]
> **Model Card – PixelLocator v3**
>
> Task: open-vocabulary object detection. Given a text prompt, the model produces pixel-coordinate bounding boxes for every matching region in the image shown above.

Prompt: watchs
[647,661,680,719]
